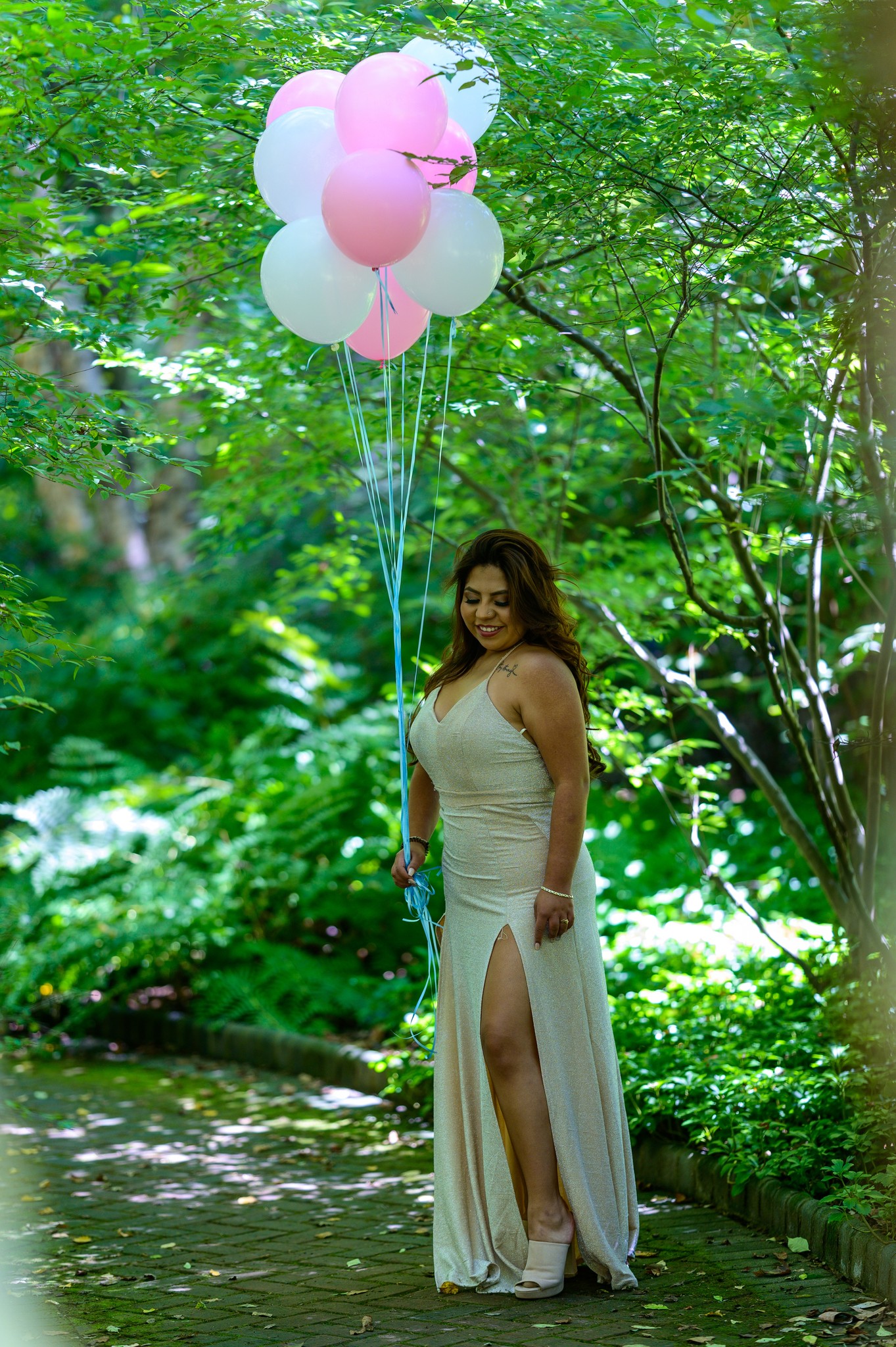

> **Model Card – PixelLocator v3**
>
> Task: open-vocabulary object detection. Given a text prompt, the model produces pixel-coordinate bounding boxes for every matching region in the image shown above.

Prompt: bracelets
[541,886,573,899]
[400,836,431,856]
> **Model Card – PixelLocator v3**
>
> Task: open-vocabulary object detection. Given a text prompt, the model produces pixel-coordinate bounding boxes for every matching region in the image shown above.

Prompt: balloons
[253,31,503,371]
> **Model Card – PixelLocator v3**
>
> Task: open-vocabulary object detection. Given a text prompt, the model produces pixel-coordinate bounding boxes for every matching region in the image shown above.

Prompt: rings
[560,919,569,924]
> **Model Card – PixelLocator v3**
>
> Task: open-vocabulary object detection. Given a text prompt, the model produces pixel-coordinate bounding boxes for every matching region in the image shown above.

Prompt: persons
[389,529,638,1299]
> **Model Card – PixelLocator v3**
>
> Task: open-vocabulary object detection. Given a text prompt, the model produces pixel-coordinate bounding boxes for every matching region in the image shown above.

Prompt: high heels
[514,1212,578,1298]
